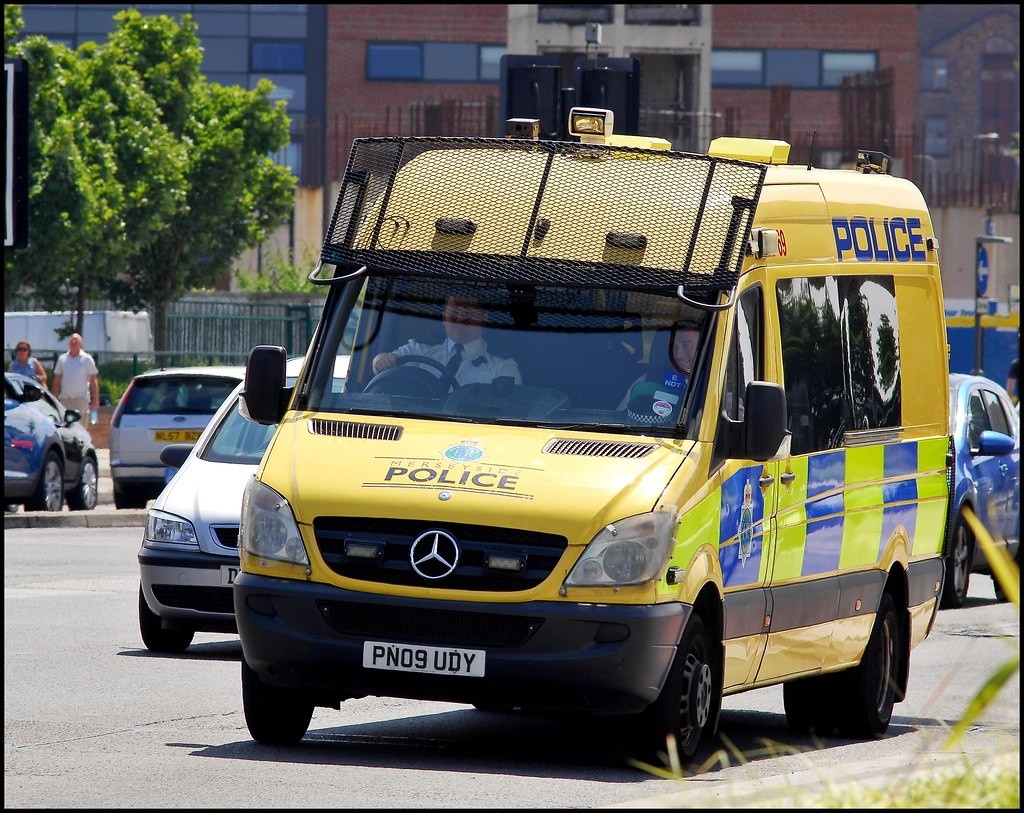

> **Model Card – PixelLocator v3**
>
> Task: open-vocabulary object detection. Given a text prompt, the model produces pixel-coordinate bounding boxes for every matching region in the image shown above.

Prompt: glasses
[16,347,29,353]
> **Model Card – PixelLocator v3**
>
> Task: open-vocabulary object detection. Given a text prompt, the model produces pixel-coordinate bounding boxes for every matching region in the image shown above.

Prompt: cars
[107,366,248,510]
[788,389,886,449]
[4,371,99,510]
[3,373,67,512]
[138,354,352,651]
[939,372,1019,609]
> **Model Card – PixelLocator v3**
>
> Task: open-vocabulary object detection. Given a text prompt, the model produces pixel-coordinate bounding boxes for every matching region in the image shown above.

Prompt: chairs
[616,327,672,410]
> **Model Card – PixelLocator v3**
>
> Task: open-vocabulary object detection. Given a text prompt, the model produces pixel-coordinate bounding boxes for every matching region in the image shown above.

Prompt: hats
[621,395,678,426]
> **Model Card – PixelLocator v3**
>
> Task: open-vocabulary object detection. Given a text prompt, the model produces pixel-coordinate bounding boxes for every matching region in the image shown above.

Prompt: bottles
[105,399,111,406]
[90,409,99,426]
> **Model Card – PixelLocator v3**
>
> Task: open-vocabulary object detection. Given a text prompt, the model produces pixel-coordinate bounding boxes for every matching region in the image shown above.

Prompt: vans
[234,107,948,769]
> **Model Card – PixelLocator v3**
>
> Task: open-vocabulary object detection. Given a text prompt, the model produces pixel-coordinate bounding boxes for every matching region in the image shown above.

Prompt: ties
[437,344,464,393]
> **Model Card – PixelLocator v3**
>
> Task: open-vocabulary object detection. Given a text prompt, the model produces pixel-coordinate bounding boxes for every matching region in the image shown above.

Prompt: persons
[373,285,522,392]
[51,333,99,429]
[8,341,47,383]
[615,320,743,423]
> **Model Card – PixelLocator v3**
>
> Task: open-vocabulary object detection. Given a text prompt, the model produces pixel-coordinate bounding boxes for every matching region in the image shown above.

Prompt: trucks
[4,311,156,367]
